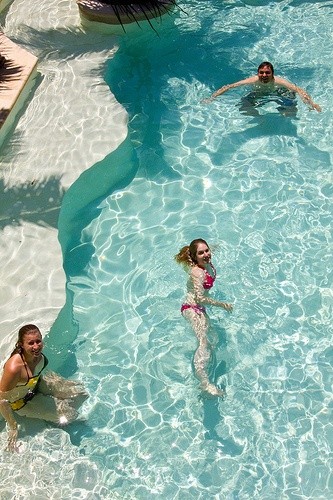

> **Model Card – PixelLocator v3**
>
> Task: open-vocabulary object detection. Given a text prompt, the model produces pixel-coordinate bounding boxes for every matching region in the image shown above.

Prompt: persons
[202,62,321,113]
[174,238,234,397]
[0,324,80,442]
